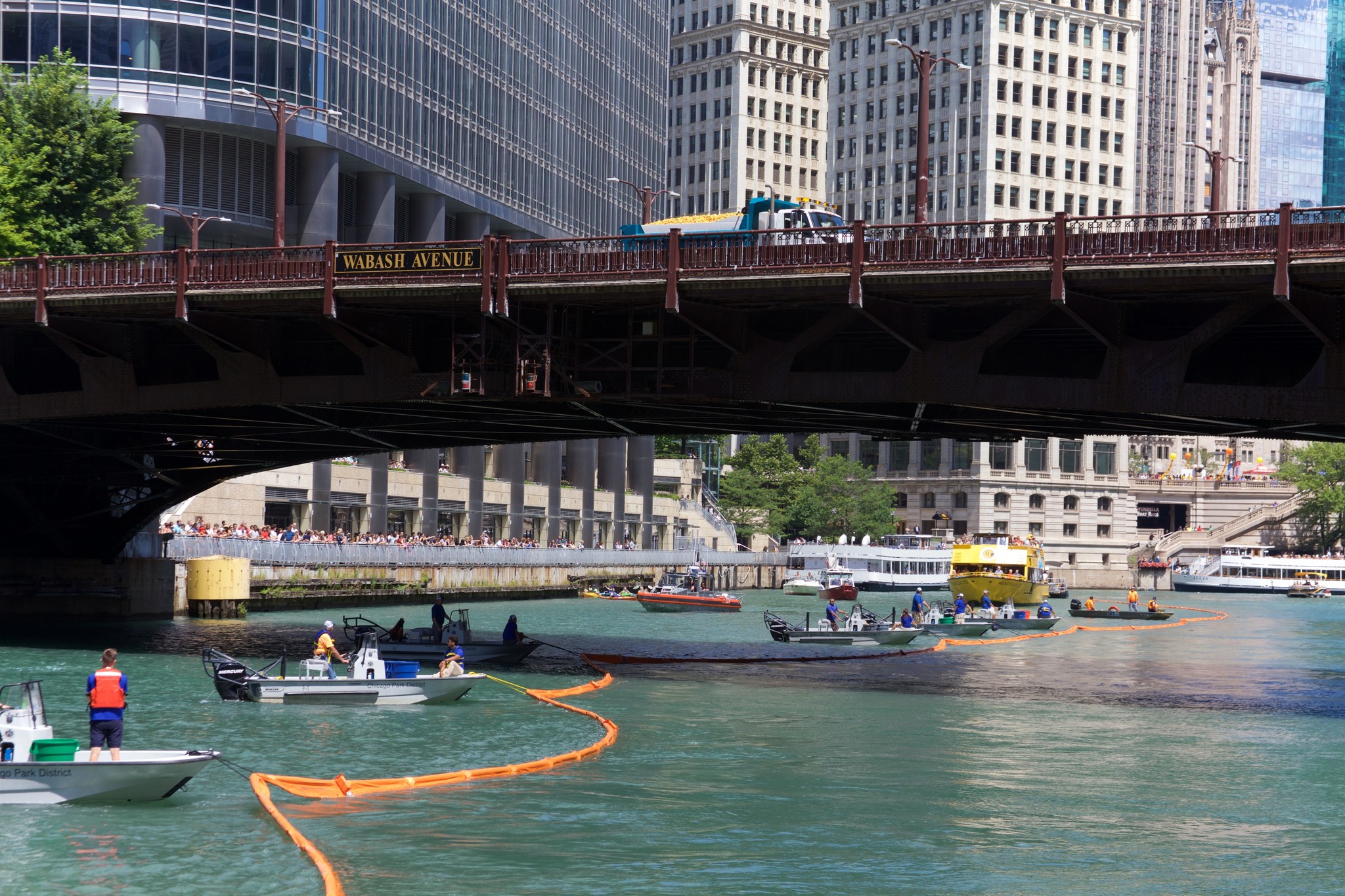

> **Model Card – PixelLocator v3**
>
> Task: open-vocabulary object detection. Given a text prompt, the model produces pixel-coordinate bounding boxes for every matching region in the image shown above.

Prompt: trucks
[619,184,882,252]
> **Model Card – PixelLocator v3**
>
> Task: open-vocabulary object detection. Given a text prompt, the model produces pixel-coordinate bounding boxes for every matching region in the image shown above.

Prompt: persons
[1085,586,1159,612]
[438,636,464,677]
[605,584,631,598]
[589,584,598,593]
[1037,600,1056,619]
[897,524,1050,580]
[891,609,917,631]
[795,570,815,581]
[638,586,649,593]
[763,537,806,565]
[314,621,351,678]
[912,588,931,624]
[432,595,453,643]
[680,495,692,509]
[161,456,638,551]
[708,505,723,529]
[86,648,127,762]
[980,590,997,619]
[1129,470,1345,586]
[691,578,709,592]
[952,594,975,625]
[503,615,528,644]
[826,599,848,631]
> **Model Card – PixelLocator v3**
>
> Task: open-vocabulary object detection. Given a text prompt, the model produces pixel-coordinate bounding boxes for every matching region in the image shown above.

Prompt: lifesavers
[1108,606,1119,611]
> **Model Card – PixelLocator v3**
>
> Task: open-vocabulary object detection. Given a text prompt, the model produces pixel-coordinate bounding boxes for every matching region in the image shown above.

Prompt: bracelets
[444,659,447,662]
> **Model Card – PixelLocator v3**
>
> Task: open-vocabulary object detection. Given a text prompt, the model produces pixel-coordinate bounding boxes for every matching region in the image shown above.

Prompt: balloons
[1160,448,1263,481]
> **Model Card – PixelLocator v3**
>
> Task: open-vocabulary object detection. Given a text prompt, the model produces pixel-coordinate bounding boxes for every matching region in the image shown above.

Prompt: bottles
[4,748,13,761]
[932,620,935,624]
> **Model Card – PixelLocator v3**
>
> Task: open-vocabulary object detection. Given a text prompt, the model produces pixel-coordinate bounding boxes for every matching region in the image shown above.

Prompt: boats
[1048,578,1069,599]
[1171,543,1345,595]
[926,595,1064,631]
[817,558,860,600]
[784,528,957,591]
[636,533,745,613]
[946,532,1051,607]
[1286,572,1334,599]
[1067,599,1175,621]
[764,602,925,645]
[598,593,638,600]
[202,631,488,706]
[782,576,822,596]
[0,677,224,806]
[583,591,603,598]
[342,607,544,666]
[853,602,999,638]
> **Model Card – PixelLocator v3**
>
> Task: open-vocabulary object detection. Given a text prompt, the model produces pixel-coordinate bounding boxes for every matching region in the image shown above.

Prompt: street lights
[147,204,232,268]
[605,177,681,225]
[882,38,973,235]
[232,85,343,256]
[890,510,895,526]
[832,507,837,545]
[1180,141,1245,227]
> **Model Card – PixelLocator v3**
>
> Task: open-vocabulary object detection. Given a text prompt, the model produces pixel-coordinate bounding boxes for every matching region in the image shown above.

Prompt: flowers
[1138,562,1170,568]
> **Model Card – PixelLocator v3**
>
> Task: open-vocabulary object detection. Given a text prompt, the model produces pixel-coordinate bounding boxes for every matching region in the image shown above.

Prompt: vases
[1141,567,1167,570]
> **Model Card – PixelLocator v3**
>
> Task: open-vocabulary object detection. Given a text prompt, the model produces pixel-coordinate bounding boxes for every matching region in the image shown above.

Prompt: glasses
[829,601,834,602]
[447,640,453,644]
[440,599,444,601]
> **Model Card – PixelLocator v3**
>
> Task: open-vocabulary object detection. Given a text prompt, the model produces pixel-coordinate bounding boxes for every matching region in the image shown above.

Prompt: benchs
[299,659,328,679]
[818,618,831,632]
[409,627,434,644]
[977,609,992,619]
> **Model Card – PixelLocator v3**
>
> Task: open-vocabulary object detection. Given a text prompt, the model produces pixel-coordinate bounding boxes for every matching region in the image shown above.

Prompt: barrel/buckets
[371,660,393,678]
[1014,611,1026,619]
[30,738,80,762]
[938,616,955,624]
[1018,610,1030,619]
[389,661,421,679]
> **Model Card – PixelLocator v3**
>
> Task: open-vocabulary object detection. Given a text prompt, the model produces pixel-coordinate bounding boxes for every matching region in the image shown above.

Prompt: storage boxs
[1070,599,1082,609]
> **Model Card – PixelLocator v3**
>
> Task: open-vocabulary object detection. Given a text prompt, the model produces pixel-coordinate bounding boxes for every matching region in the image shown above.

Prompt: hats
[1153,596,1156,599]
[323,620,335,628]
[984,590,990,594]
[957,593,964,597]
[917,587,922,591]
[1043,600,1048,603]
[436,595,446,600]
[508,615,517,621]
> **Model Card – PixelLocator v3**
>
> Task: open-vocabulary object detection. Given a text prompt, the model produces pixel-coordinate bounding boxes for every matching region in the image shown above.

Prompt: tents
[1243,463,1278,480]
